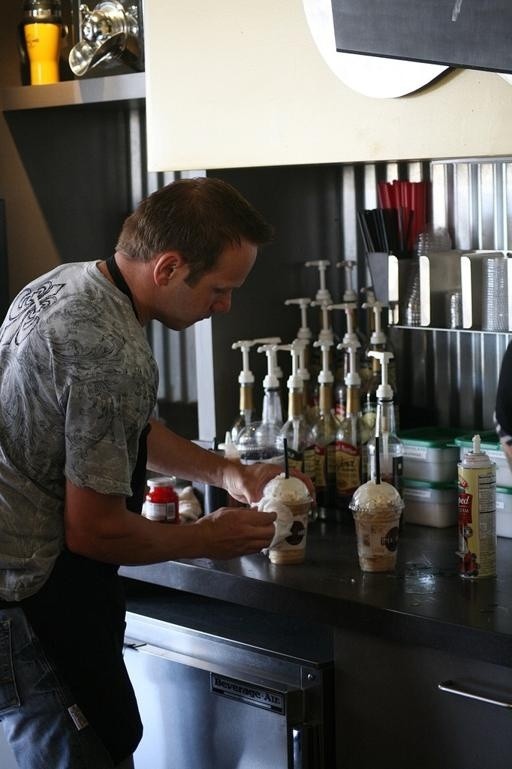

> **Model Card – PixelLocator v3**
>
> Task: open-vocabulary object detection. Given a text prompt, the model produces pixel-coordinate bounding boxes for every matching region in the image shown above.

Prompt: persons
[0,177,319,768]
[492,339,512,469]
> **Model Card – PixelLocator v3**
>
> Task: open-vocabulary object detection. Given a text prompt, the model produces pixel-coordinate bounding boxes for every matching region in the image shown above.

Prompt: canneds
[144,476,180,524]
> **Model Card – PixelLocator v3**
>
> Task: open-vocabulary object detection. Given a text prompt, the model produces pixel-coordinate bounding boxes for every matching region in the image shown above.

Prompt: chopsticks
[378,181,427,235]
[353,206,414,252]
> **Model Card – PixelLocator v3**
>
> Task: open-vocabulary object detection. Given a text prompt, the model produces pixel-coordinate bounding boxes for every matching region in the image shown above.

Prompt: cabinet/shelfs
[332,628,511,769]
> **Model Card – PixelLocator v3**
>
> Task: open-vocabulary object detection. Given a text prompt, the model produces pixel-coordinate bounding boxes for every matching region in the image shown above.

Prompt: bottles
[219,430,237,459]
[308,297,339,343]
[289,338,314,393]
[230,371,254,445]
[367,384,405,534]
[287,374,314,522]
[364,344,399,434]
[204,438,229,517]
[314,369,341,519]
[338,372,375,526]
[261,376,285,428]
[312,340,336,404]
[336,342,361,404]
[457,452,497,580]
[333,298,361,343]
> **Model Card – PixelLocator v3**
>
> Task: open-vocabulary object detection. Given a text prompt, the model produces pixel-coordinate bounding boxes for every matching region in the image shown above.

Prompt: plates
[494,71,512,86]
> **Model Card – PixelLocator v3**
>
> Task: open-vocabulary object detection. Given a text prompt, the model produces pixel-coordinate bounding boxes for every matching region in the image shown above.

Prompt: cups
[402,227,450,328]
[20,0,67,84]
[270,472,314,565]
[479,256,509,334]
[236,424,285,466]
[348,479,406,573]
[443,287,464,329]
